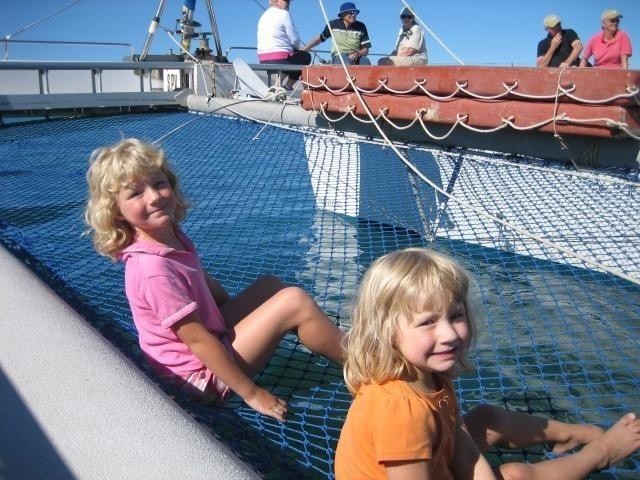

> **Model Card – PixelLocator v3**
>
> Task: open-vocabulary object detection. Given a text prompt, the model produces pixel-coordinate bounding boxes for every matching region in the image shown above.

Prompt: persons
[578,9,632,70]
[299,2,372,65]
[536,15,592,69]
[256,0,311,90]
[334,247,640,480]
[377,7,428,66]
[78,138,352,422]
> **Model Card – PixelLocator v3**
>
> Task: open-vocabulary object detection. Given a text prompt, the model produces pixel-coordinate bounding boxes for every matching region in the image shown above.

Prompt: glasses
[347,11,358,15]
[400,14,414,19]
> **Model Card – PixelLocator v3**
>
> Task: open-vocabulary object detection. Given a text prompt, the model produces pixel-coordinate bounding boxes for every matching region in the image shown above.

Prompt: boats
[287,209,640,466]
[160,1,640,287]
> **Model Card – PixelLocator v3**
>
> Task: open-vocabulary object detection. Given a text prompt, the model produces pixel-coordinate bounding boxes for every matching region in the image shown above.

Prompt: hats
[601,9,624,22]
[543,14,562,31]
[338,2,360,18]
